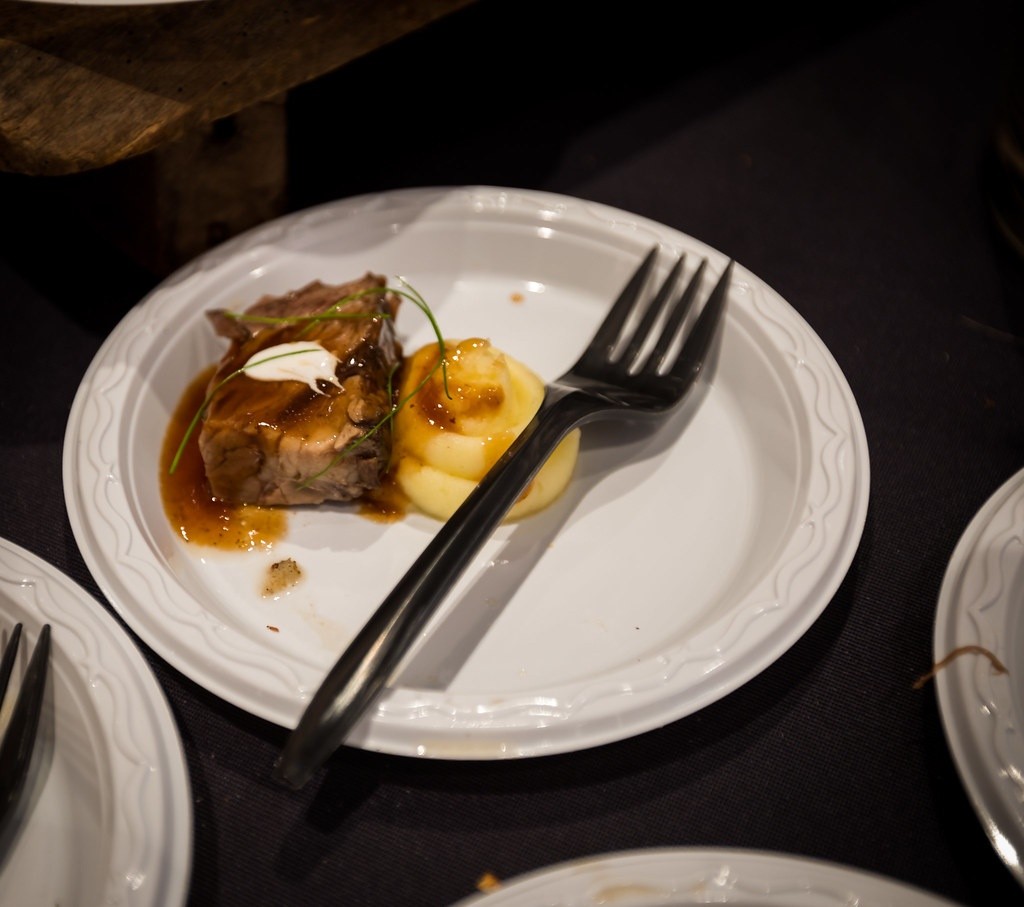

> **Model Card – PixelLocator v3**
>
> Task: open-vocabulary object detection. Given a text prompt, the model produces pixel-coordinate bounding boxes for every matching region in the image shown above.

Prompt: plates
[932,465,1024,888]
[0,536,195,907]
[440,845,954,907]
[62,185,872,762]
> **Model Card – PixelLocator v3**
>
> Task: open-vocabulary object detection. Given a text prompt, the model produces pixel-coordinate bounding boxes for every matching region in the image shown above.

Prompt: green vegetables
[167,274,454,491]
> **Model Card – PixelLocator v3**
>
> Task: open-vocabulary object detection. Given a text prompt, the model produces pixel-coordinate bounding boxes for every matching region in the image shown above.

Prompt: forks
[0,622,50,837]
[271,243,736,793]
